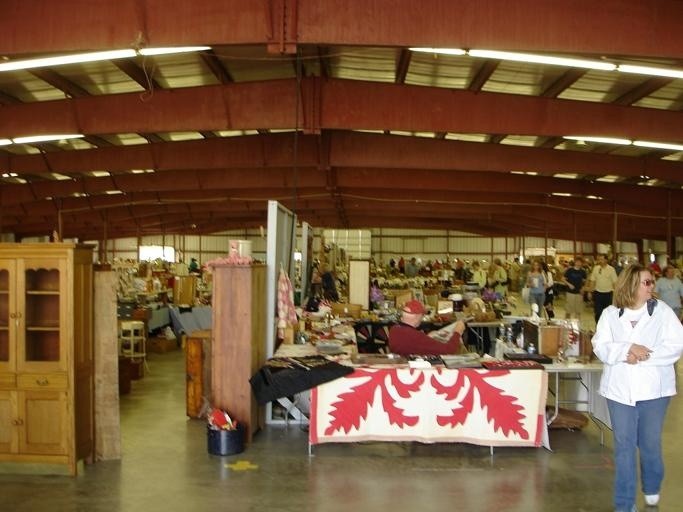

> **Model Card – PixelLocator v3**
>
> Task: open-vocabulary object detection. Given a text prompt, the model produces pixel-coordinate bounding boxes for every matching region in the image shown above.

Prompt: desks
[266,295,609,457]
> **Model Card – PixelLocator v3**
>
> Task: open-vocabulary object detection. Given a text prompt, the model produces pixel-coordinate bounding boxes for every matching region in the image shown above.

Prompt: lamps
[409,46,683,79]
[562,136,683,151]
[0,44,213,74]
[0,134,85,146]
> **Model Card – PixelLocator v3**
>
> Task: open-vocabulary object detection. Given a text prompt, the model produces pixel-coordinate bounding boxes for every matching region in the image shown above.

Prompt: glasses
[644,280,654,285]
[599,268,602,274]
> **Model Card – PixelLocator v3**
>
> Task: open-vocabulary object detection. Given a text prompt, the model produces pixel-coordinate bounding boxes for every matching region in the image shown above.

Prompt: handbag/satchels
[522,284,531,304]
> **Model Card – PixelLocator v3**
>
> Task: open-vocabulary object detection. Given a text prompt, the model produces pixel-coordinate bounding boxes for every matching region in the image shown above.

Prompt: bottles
[507,327,513,344]
[528,343,535,356]
[499,324,505,341]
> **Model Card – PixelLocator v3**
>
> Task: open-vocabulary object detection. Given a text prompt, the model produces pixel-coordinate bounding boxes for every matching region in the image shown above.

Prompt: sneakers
[645,495,659,505]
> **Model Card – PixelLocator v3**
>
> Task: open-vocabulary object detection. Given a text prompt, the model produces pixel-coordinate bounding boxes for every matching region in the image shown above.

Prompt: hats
[402,300,428,315]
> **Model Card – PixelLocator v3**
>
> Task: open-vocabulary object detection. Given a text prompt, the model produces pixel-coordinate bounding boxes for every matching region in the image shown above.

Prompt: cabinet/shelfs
[0,242,96,478]
[110,257,265,443]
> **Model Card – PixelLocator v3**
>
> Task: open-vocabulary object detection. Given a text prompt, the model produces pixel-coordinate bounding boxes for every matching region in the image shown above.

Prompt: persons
[387,300,466,355]
[589,262,682,511]
[389,253,683,329]
[188,257,200,271]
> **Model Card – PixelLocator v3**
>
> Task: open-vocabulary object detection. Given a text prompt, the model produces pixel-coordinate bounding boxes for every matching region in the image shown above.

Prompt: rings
[645,353,649,357]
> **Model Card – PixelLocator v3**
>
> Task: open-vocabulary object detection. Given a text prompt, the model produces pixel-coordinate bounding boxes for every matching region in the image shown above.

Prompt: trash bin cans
[207,423,245,456]
[119,357,132,395]
